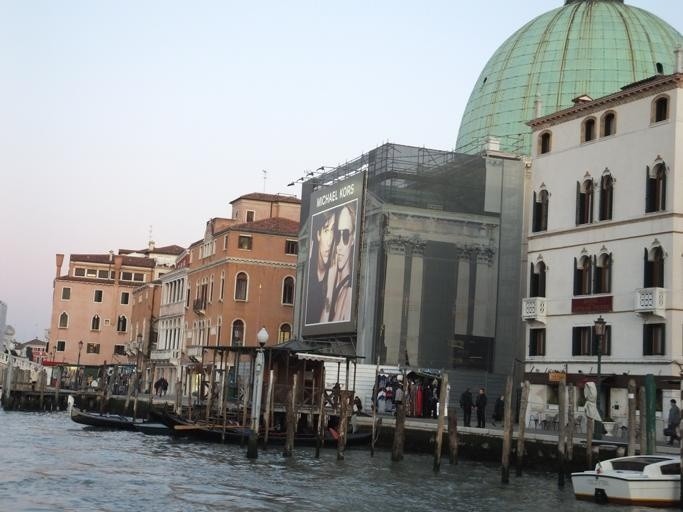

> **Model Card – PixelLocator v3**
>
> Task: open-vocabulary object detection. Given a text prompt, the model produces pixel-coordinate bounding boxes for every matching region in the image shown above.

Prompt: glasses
[333,227,355,245]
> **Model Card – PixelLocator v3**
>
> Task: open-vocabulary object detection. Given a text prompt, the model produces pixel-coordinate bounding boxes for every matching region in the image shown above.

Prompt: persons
[29,366,40,390]
[458,386,474,426]
[663,398,681,447]
[324,202,353,323]
[470,388,488,427]
[490,394,507,428]
[352,395,363,411]
[61,367,168,396]
[302,210,337,323]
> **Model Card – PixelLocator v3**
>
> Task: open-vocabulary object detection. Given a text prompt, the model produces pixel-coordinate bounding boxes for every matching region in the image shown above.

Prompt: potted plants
[601,417,617,437]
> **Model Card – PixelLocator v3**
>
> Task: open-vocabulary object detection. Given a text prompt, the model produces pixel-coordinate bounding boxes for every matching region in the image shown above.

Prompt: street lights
[135,333,143,396]
[73,340,84,390]
[593,313,607,416]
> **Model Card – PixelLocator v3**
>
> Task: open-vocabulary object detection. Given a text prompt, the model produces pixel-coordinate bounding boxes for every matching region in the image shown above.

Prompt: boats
[571,454,683,508]
[71,408,191,436]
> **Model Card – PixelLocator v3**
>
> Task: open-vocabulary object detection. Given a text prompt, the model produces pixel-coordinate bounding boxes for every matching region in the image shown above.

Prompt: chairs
[530,411,582,433]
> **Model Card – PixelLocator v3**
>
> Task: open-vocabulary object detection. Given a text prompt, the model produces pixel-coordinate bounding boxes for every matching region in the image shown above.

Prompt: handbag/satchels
[663,425,676,436]
